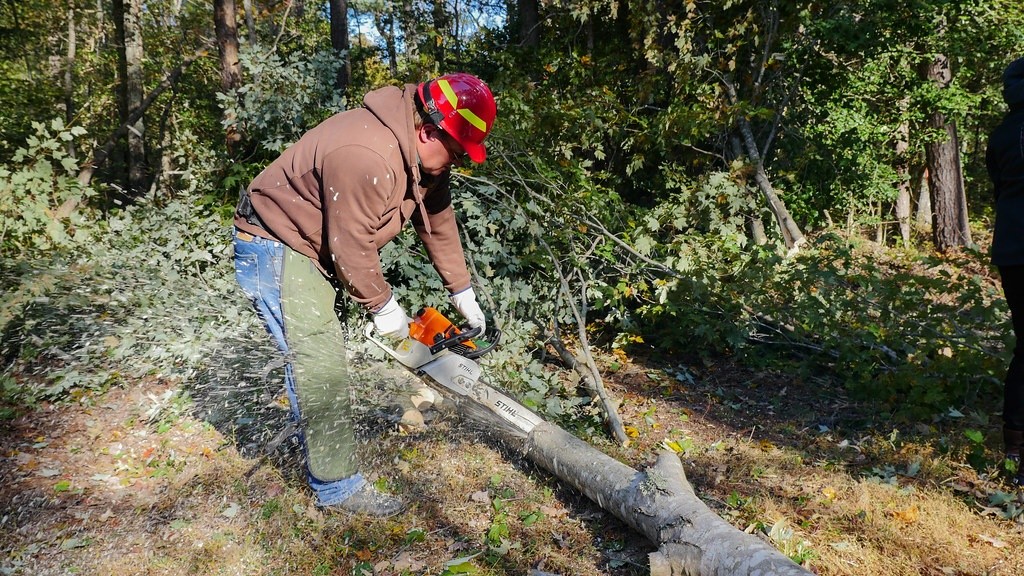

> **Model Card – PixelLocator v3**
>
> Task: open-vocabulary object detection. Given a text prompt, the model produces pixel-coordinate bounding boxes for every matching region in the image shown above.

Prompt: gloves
[373,292,413,340]
[449,286,486,339]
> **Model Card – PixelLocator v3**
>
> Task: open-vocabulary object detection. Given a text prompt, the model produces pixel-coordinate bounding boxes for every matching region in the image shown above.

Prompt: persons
[231,72,497,520]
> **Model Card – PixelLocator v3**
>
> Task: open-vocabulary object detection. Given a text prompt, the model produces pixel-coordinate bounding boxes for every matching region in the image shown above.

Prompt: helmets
[418,74,496,164]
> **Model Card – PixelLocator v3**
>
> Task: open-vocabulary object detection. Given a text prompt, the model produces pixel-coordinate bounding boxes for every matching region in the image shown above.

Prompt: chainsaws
[361,305,545,434]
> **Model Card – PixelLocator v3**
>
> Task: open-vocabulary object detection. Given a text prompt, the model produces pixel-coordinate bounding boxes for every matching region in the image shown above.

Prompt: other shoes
[318,483,405,517]
